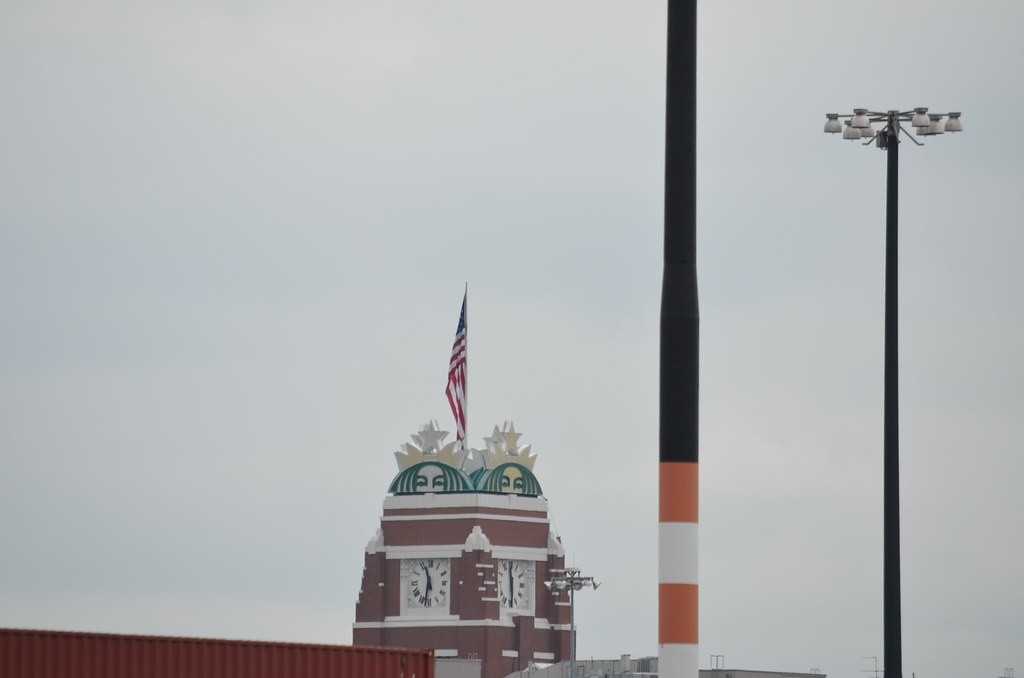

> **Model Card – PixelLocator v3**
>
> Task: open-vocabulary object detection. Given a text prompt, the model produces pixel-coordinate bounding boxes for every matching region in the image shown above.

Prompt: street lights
[824,107,962,678]
[544,568,602,662]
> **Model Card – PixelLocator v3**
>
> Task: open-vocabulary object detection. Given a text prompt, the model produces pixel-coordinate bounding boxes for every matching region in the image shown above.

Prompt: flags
[444,292,468,448]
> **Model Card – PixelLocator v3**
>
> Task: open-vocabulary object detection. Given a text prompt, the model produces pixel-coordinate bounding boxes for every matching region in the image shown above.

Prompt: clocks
[497,560,530,609]
[405,559,451,610]
